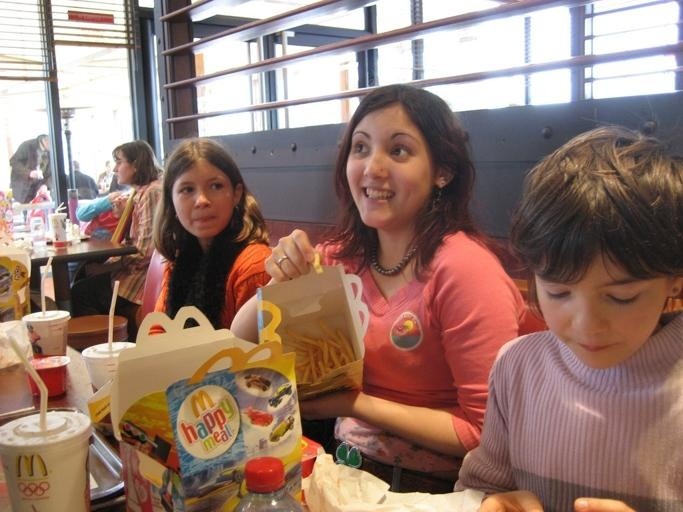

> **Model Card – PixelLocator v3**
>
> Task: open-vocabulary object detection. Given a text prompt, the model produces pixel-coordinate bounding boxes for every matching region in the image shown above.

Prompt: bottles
[0,412,96,511]
[65,218,82,244]
[231,455,304,511]
[30,216,46,250]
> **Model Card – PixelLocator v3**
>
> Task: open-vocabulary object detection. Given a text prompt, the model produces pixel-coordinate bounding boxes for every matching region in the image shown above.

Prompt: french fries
[282,321,357,384]
[307,254,323,275]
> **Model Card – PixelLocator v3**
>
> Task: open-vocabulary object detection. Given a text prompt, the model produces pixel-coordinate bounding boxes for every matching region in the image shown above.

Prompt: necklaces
[369,230,421,275]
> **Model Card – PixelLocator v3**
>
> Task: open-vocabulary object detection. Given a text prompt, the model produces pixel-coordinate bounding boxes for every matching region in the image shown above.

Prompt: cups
[49,213,67,251]
[67,189,79,224]
[24,357,67,400]
[21,308,67,358]
[82,344,133,394]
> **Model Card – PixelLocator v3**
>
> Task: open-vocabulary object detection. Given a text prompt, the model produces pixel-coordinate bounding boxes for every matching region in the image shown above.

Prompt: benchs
[149,88,681,476]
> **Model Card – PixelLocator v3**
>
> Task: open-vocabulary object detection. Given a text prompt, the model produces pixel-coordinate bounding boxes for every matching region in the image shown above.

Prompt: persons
[229,84,523,491]
[453,123,683,512]
[10,134,166,315]
[156,138,276,331]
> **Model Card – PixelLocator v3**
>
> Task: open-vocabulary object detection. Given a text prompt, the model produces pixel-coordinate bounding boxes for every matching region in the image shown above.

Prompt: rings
[277,255,287,265]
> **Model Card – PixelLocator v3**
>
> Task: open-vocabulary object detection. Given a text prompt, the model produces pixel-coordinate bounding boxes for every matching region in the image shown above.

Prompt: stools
[63,316,130,354]
